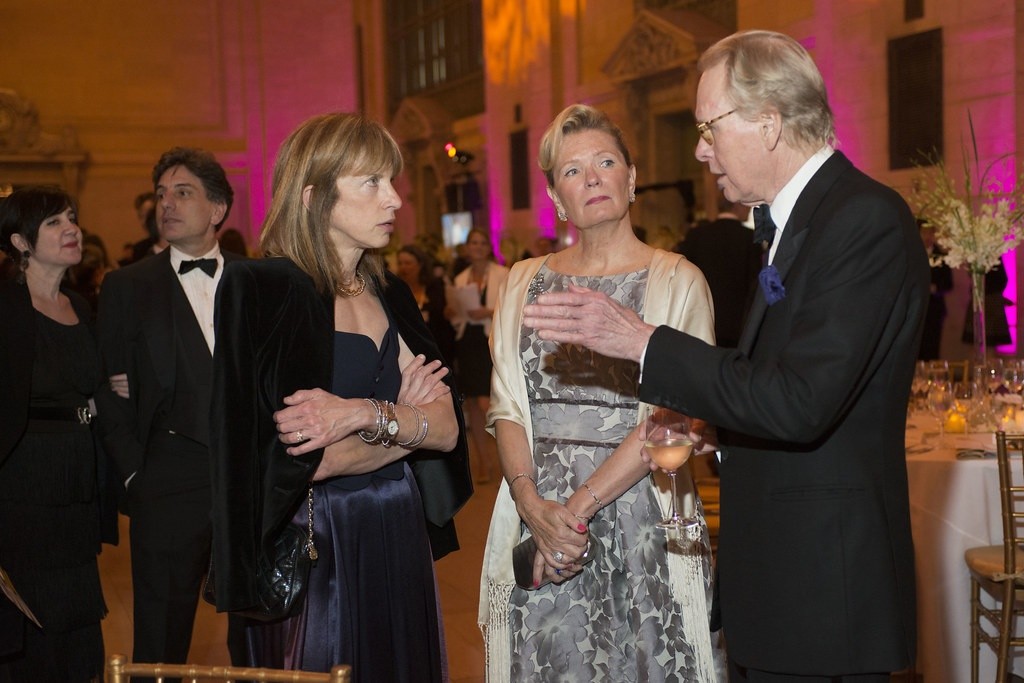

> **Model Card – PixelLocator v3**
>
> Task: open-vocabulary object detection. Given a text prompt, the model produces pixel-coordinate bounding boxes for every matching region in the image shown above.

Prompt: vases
[970,270,989,422]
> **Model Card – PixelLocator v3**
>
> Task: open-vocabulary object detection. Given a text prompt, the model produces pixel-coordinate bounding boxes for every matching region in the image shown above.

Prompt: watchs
[379,398,399,439]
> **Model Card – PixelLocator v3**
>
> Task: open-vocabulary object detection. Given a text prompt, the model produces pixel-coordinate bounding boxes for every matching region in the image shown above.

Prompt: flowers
[897,107,1024,315]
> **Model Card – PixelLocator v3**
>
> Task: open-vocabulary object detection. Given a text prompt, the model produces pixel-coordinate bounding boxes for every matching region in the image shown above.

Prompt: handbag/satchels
[202,551,311,624]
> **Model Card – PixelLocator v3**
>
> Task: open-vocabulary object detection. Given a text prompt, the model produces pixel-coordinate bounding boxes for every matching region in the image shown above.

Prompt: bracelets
[408,404,428,450]
[581,482,605,507]
[397,402,420,446]
[509,472,538,500]
[359,395,399,446]
[565,501,594,520]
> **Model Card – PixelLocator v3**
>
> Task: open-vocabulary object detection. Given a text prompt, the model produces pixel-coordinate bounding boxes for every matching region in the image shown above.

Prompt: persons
[959,256,1014,357]
[97,145,233,683]
[472,103,717,683]
[214,113,475,683]
[917,216,953,361]
[393,230,561,482]
[520,31,931,683]
[682,187,764,354]
[0,173,133,682]
[61,194,240,322]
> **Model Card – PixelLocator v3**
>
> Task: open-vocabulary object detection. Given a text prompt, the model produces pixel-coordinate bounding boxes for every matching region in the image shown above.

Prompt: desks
[905,399,1024,683]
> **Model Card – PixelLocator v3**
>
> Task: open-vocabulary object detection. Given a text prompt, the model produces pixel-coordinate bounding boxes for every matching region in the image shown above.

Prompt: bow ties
[178,259,218,277]
[752,204,776,244]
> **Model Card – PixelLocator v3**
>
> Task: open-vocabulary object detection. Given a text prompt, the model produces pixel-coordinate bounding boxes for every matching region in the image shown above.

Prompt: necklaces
[342,270,366,298]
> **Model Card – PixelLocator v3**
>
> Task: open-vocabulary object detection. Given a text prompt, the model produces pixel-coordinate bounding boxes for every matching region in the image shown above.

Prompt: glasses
[697,109,737,146]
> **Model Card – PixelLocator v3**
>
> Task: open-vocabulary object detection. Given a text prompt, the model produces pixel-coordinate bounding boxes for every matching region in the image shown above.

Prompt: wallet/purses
[512,532,596,591]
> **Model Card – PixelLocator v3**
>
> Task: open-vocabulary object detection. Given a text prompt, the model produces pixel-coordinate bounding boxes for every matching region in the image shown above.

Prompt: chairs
[964,430,1024,683]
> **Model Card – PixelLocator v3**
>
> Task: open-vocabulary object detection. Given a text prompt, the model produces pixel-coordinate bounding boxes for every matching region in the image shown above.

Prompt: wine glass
[910,358,1024,448]
[646,405,698,527]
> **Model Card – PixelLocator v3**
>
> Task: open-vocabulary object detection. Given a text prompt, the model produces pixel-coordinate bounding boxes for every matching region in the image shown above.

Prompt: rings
[296,430,302,442]
[550,551,563,564]
[554,569,560,574]
